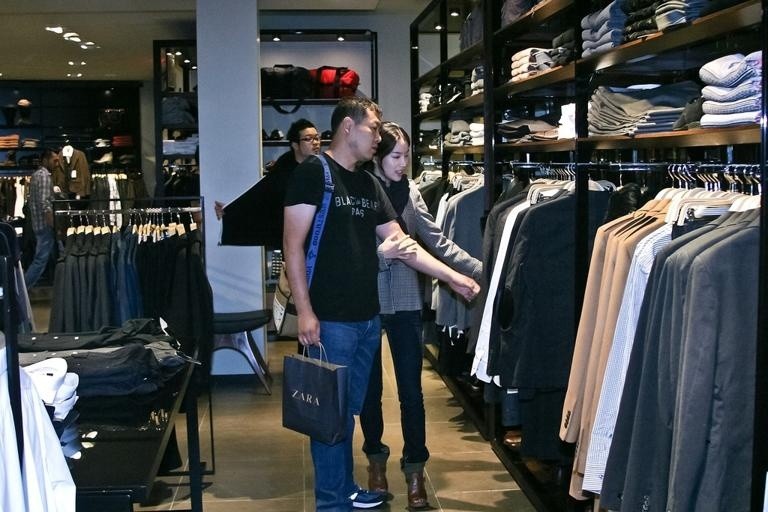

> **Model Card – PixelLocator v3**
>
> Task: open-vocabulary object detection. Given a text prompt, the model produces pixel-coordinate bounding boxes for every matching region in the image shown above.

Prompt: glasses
[300,136,319,142]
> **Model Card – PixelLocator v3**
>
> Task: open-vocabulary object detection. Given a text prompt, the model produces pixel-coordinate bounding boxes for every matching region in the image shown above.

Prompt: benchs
[211,309,273,394]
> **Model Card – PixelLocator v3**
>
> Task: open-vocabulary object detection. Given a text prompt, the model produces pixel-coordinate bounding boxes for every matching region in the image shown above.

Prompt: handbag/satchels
[261,64,359,114]
[282,342,347,446]
[273,266,299,338]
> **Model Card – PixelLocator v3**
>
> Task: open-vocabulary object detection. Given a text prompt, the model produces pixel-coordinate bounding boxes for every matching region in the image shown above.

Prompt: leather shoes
[367,461,390,492]
[400,472,427,508]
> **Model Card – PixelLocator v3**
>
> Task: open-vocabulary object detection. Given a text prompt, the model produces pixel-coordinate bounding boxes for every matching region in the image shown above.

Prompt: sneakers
[349,490,383,509]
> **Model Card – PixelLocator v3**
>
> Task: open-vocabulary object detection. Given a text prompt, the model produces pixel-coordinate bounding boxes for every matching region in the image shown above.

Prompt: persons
[56,143,92,227]
[213,116,322,250]
[358,118,483,510]
[281,95,482,511]
[25,149,58,292]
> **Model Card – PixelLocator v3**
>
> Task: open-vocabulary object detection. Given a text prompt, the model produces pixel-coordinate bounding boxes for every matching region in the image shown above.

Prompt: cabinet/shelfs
[153,31,378,197]
[0,80,144,172]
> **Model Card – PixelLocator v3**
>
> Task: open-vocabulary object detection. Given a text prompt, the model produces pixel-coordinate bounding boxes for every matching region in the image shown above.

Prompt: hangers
[64,206,197,243]
[0,168,129,182]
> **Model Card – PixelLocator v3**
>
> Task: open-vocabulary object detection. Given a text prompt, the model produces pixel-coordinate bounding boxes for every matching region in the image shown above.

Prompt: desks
[59,339,202,511]
